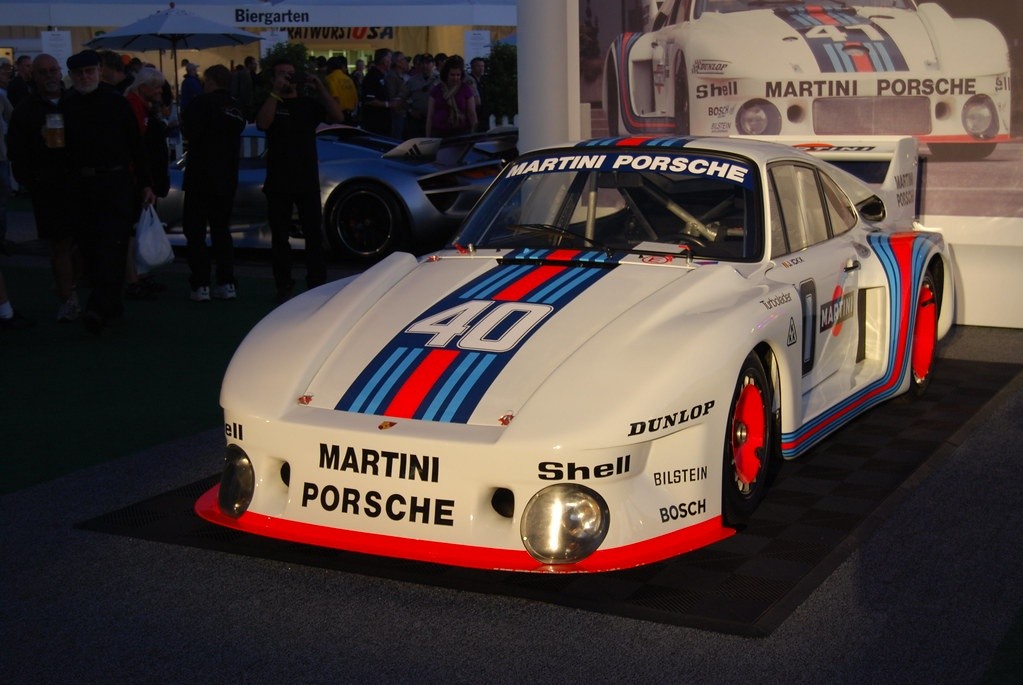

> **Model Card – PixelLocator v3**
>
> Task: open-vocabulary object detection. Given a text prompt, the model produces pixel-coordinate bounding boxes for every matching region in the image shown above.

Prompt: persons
[352,48,491,141]
[255,61,344,301]
[176,57,268,302]
[0,50,174,335]
[305,55,364,126]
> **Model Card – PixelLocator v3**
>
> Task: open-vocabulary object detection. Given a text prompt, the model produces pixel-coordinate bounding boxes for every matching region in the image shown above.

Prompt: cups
[46,114,64,147]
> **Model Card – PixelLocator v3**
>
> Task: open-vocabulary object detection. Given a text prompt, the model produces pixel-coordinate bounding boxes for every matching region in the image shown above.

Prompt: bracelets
[384,101,390,110]
[268,91,283,102]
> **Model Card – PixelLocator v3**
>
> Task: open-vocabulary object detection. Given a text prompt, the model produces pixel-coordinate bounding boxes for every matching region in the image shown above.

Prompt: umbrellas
[83,1,266,117]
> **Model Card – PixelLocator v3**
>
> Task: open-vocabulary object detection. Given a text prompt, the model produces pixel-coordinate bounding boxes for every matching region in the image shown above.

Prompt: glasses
[71,66,97,75]
[32,66,60,75]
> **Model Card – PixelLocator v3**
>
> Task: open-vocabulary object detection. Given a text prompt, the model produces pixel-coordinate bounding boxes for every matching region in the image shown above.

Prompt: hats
[416,53,433,65]
[186,64,199,72]
[67,54,99,69]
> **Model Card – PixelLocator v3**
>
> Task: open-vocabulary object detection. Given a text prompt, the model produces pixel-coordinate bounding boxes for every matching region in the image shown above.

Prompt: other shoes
[73,280,93,310]
[305,264,327,288]
[54,297,82,323]
[0,309,35,329]
[276,277,296,289]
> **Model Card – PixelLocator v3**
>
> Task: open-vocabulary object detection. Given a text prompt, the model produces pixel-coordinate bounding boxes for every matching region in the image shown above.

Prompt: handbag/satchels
[129,202,175,267]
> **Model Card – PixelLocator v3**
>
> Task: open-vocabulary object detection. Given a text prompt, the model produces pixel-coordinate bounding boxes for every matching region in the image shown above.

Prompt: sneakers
[189,286,211,301]
[211,284,237,299]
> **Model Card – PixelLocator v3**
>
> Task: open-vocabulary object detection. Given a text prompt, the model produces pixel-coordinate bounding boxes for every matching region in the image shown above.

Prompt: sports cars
[154,124,520,263]
[196,134,953,575]
[604,0,1011,160]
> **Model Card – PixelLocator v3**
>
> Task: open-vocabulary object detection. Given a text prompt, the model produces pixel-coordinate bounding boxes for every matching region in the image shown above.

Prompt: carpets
[75,355,1022,640]
